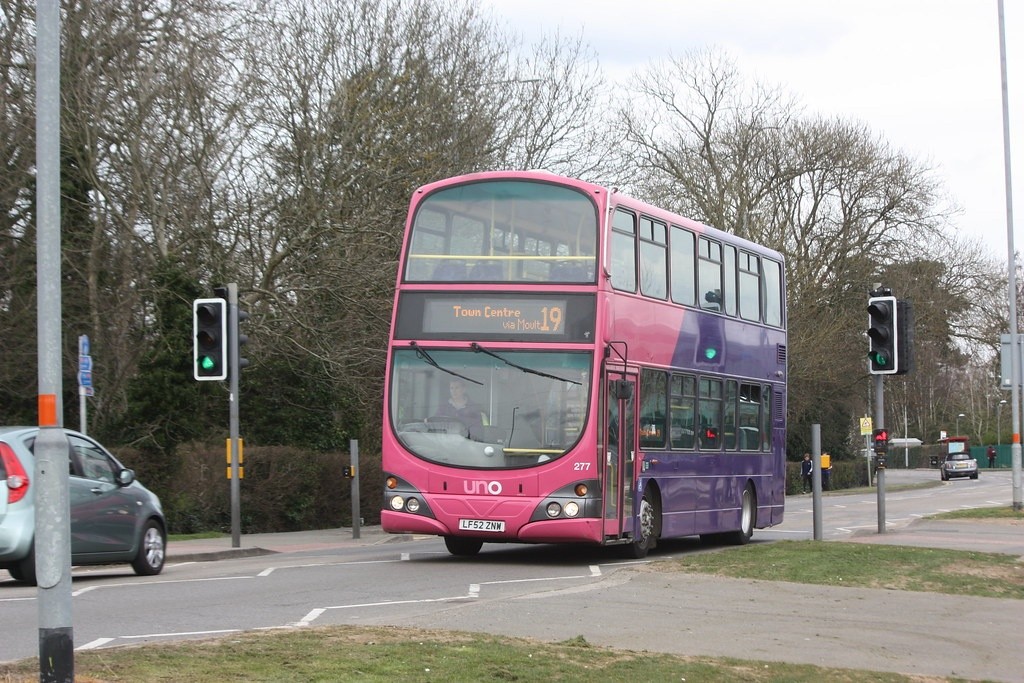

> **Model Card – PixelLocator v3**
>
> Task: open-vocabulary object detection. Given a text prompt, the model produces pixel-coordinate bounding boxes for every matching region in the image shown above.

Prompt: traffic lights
[706,427,717,445]
[194,298,226,380]
[874,428,890,457]
[866,296,897,374]
[343,466,352,479]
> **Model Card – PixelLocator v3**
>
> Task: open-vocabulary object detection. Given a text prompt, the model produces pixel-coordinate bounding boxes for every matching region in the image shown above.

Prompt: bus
[376,168,790,555]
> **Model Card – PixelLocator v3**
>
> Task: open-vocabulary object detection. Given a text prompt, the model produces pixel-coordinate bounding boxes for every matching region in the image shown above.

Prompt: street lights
[956,413,965,437]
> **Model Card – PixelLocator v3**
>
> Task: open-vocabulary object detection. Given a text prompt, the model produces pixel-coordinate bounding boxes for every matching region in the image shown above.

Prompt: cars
[0,424,168,585]
[940,452,978,481]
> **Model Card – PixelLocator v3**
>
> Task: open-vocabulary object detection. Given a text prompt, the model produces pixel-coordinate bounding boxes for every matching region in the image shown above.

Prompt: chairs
[433,263,502,278]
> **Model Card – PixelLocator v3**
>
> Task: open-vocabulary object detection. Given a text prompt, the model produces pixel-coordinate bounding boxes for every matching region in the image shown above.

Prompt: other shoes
[803,492,806,494]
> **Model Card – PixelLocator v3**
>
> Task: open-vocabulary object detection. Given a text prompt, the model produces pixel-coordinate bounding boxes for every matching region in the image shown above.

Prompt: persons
[987,444,996,468]
[434,378,484,442]
[800,451,832,494]
